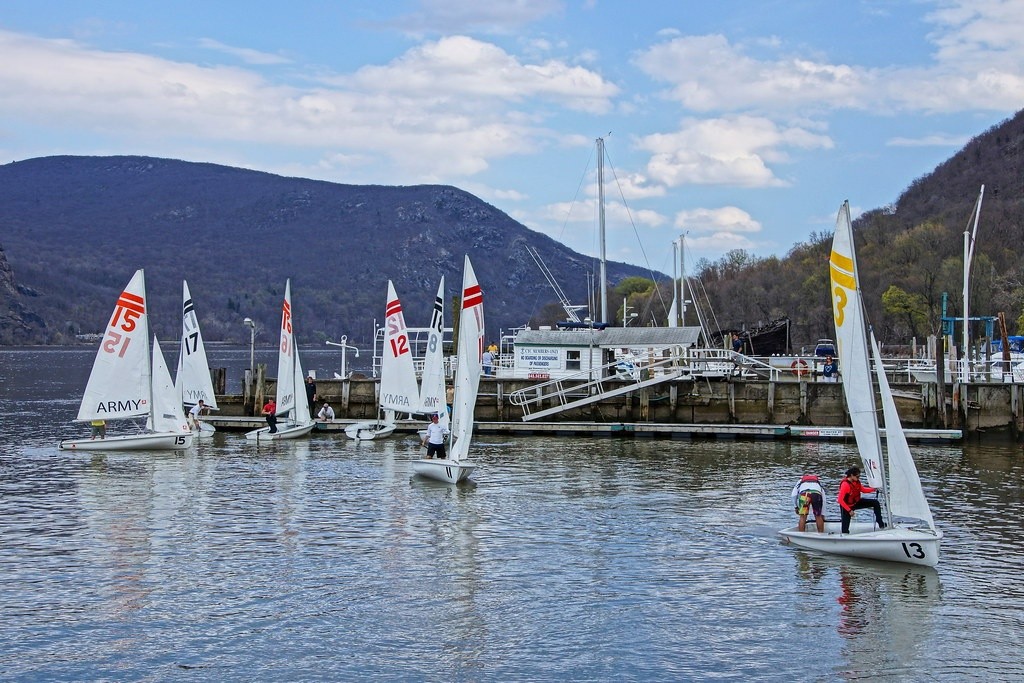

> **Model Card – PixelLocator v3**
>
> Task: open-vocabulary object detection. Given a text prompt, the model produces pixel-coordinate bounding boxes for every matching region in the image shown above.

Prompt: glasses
[855,475,861,478]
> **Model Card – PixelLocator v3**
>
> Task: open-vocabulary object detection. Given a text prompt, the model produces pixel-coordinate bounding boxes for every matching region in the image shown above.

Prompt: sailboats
[60,268,194,450]
[775,199,944,570]
[413,136,796,378]
[344,278,426,441]
[416,273,454,444]
[411,252,486,484]
[244,277,316,441]
[908,183,1024,383]
[174,279,221,438]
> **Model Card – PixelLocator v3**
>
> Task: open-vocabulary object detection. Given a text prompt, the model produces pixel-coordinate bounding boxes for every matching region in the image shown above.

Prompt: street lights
[244,317,255,387]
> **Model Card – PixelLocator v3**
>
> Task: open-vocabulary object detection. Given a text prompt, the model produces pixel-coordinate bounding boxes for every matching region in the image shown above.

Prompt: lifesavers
[790,360,808,376]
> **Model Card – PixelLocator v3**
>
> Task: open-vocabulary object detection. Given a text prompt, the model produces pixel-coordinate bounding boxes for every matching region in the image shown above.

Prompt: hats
[322,403,328,408]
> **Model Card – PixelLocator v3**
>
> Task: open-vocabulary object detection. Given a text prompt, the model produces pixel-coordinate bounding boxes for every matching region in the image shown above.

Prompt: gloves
[795,506,799,514]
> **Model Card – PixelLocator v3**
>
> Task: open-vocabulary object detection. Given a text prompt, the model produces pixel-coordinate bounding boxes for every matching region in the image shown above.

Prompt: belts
[189,412,194,415]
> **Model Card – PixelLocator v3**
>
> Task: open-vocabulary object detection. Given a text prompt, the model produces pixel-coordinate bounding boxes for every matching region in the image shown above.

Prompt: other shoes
[879,522,887,528]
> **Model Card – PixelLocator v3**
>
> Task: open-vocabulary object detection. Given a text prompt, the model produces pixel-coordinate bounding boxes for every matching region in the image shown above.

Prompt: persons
[447,406,450,416]
[91,420,105,440]
[262,397,277,433]
[306,377,317,420]
[188,399,210,432]
[823,355,839,382]
[483,350,494,378]
[838,466,887,535]
[791,476,826,533]
[421,414,450,460]
[729,334,742,376]
[318,403,334,423]
[488,342,497,353]
